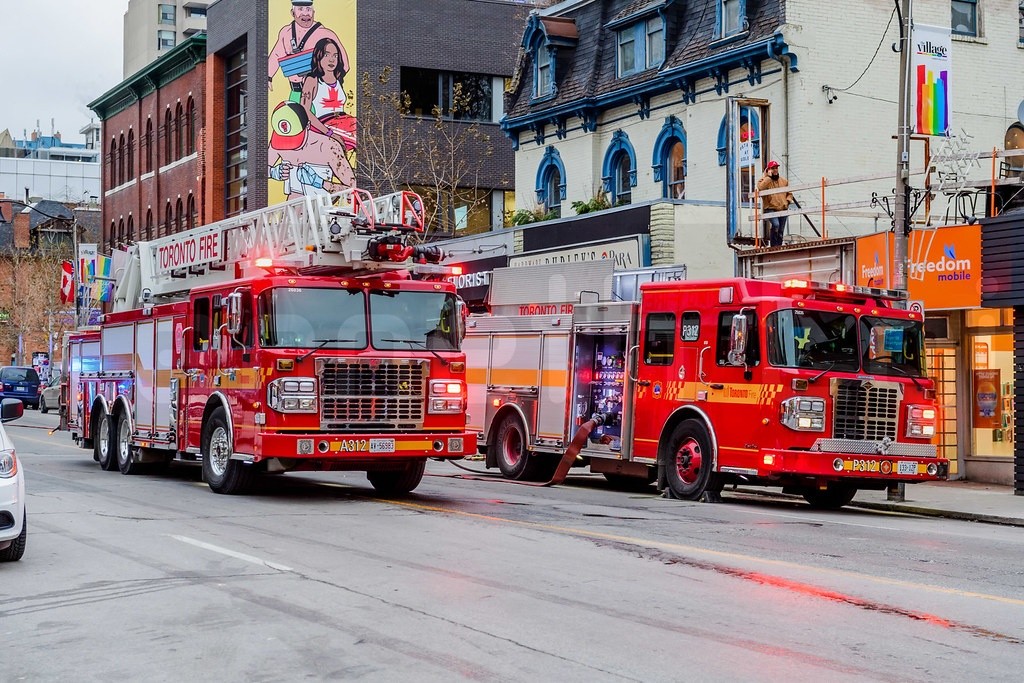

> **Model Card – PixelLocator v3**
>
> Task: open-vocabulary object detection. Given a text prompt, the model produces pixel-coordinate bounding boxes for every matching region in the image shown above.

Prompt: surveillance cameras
[143,289,150,299]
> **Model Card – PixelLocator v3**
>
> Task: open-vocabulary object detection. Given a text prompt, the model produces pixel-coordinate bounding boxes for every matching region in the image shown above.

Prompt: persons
[757,161,794,246]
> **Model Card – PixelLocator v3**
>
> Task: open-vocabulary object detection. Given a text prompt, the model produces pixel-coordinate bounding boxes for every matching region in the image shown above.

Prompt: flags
[71,255,112,302]
[60,259,74,305]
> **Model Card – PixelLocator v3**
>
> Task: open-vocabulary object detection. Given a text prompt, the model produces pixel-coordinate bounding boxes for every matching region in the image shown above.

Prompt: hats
[768,161,780,168]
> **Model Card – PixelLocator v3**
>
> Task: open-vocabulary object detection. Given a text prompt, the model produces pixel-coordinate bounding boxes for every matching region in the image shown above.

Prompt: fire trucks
[64,189,469,498]
[457,258,951,501]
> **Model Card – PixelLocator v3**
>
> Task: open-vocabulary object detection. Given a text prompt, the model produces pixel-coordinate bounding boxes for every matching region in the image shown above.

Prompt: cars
[39,374,65,413]
[0,398,27,561]
[0,365,42,409]
